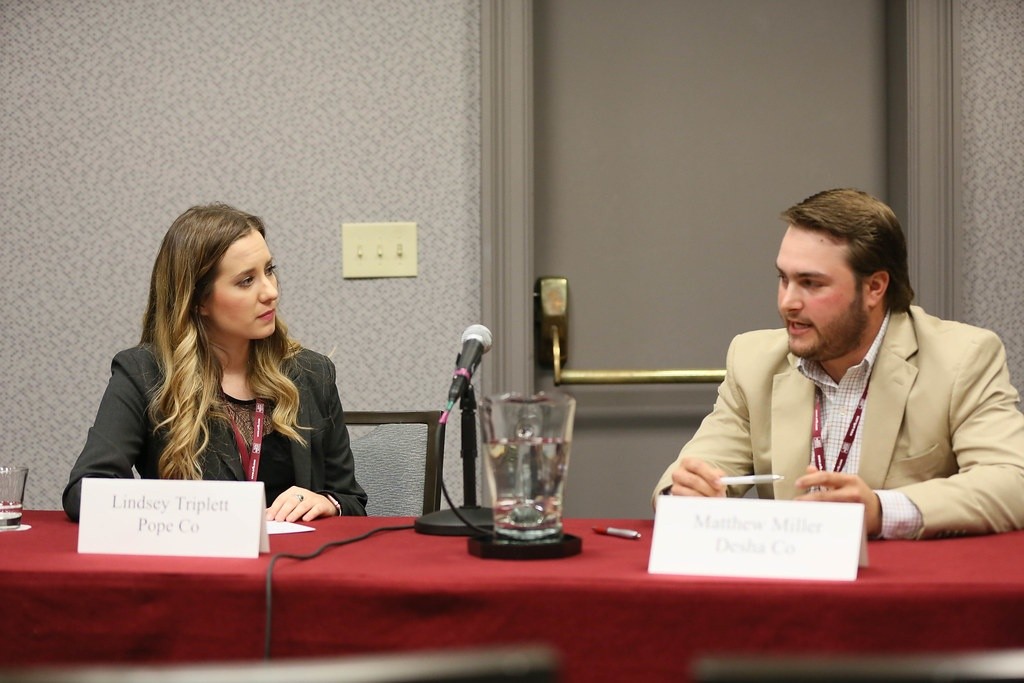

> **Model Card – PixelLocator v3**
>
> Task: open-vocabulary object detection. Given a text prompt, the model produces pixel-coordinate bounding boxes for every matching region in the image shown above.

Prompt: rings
[295,494,304,502]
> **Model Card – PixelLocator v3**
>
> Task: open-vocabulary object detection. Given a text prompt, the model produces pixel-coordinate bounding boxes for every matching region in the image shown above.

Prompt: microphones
[447,323,494,413]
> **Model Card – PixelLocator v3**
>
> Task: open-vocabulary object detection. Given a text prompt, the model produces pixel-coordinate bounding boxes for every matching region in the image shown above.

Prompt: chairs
[345,410,448,515]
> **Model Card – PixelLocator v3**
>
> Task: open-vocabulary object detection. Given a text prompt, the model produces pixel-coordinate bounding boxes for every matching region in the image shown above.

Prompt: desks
[0,510,1024,683]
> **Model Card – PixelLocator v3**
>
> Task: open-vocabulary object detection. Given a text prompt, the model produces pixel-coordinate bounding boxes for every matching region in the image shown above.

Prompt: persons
[62,201,368,524]
[650,187,1023,540]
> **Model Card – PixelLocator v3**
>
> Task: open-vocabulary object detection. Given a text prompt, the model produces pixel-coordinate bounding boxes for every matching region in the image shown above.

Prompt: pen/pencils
[721,473,786,486]
[590,524,641,539]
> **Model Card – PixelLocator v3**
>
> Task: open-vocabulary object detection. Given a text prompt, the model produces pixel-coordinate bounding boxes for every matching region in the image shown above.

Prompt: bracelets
[320,493,342,517]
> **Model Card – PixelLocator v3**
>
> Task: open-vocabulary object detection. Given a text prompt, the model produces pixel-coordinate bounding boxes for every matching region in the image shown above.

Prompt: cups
[479,391,575,540]
[0,466,29,530]
